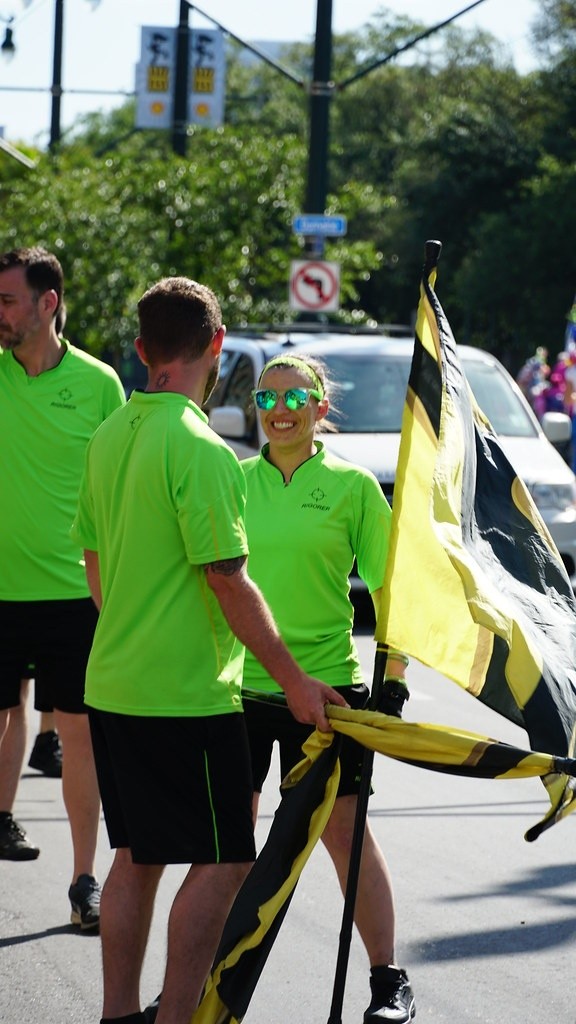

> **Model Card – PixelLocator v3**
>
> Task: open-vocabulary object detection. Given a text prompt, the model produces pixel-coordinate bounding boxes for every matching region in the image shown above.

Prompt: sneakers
[363,967,416,1024]
[68,874,102,929]
[28,734,62,777]
[0,813,40,861]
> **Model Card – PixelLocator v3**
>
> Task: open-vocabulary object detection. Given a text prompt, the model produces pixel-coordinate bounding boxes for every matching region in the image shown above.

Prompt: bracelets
[388,656,408,665]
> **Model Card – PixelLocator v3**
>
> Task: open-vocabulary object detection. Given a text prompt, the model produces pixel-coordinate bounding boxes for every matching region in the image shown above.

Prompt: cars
[201,313,576,608]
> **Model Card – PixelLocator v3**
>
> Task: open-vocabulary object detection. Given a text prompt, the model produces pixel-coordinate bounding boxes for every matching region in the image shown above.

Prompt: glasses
[251,387,323,411]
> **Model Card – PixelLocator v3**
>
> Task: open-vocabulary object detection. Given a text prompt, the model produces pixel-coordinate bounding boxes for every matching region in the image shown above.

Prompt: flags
[191,261,576,1024]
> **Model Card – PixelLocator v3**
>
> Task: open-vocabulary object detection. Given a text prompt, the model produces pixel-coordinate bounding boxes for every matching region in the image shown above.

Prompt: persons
[237,353,416,1024]
[73,278,351,1024]
[0,244,129,927]
